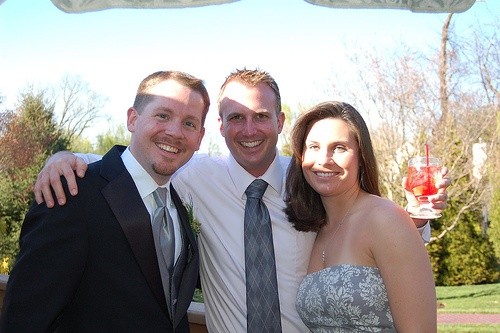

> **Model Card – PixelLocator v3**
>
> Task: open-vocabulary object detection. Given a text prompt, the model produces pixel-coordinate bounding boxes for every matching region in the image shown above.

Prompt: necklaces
[318,187,362,264]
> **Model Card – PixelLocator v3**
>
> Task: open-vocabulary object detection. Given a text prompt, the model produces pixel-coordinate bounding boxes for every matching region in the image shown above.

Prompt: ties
[244,179,282,333]
[152,187,175,324]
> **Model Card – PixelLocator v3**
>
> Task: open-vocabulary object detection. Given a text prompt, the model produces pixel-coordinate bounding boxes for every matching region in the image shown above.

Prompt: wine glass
[405,157,445,220]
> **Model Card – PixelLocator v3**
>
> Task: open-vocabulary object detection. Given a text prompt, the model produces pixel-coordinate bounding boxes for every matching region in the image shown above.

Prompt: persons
[34,69,453,333]
[281,99,440,333]
[0,69,212,333]
